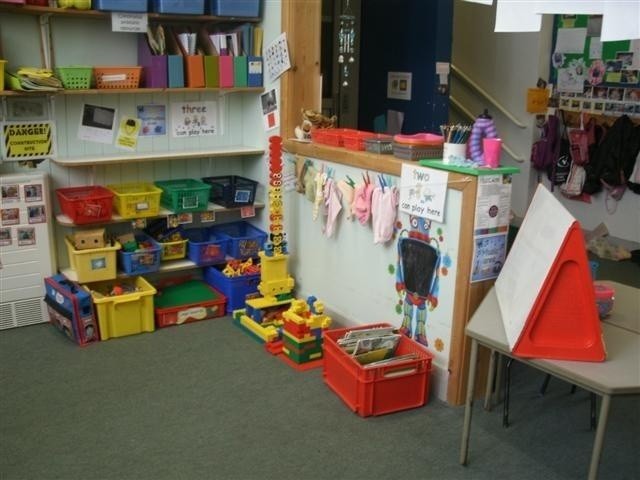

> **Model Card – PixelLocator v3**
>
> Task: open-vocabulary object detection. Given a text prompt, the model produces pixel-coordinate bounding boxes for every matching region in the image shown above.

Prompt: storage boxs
[96,1,265,20]
[149,282,225,329]
[208,260,291,320]
[323,321,434,416]
[87,274,157,343]
[63,220,267,281]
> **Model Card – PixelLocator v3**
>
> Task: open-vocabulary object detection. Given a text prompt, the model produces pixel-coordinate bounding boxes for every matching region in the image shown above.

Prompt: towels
[295,165,397,242]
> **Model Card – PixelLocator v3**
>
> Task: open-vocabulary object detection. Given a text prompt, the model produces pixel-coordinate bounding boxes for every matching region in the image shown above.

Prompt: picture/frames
[0,120,58,162]
[0,182,47,247]
[387,71,412,100]
[135,102,168,138]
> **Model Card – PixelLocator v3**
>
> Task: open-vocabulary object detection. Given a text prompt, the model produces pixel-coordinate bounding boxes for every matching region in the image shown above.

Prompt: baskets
[52,63,143,91]
[112,219,269,276]
[311,126,446,162]
[54,174,259,225]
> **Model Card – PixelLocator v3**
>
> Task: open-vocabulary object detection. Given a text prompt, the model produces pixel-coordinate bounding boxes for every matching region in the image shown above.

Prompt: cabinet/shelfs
[18,3,267,286]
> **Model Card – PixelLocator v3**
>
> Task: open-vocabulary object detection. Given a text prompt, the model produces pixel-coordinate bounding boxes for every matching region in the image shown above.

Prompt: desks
[460,272,640,479]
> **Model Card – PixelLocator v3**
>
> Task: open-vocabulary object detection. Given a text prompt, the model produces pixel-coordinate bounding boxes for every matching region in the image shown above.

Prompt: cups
[593,284,614,319]
[443,142,466,164]
[483,138,503,167]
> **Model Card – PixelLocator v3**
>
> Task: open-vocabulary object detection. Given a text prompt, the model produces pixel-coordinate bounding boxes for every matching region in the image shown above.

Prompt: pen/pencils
[440,123,471,143]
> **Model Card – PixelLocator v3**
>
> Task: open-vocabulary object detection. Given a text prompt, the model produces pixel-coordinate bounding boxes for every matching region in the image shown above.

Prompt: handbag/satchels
[530,107,639,215]
